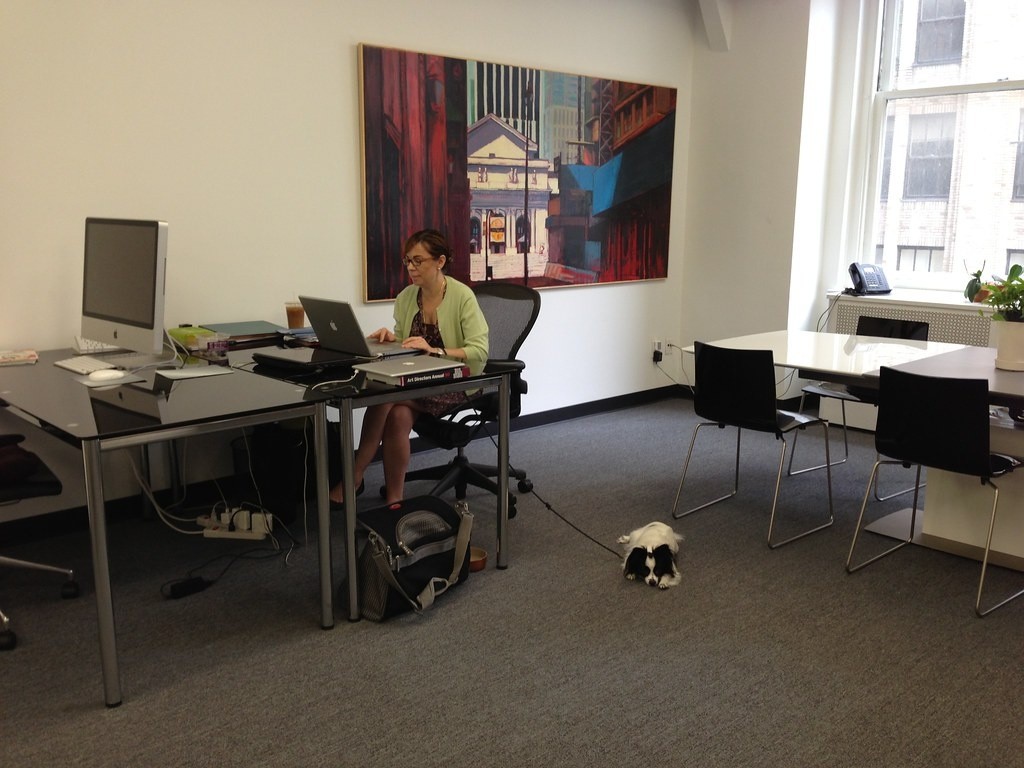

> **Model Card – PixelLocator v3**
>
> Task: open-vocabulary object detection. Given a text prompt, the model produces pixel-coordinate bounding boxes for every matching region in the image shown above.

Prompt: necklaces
[423,279,444,326]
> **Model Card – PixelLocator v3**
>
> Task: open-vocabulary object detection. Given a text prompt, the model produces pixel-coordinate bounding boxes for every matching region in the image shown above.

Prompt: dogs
[616,521,684,589]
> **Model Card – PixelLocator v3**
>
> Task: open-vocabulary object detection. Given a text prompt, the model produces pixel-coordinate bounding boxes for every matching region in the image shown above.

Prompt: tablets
[352,355,465,377]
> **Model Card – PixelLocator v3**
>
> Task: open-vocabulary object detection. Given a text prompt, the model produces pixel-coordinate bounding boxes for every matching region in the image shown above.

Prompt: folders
[198,320,288,343]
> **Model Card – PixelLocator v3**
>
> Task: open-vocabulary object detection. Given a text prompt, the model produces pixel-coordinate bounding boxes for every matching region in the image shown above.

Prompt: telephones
[848,263,891,294]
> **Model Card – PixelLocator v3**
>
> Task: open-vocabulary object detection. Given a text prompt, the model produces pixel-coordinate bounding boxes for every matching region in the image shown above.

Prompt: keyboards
[53,356,118,375]
[76,335,118,354]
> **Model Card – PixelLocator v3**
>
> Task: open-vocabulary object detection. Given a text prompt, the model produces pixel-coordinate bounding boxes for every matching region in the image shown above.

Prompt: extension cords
[196,515,228,528]
[202,525,267,540]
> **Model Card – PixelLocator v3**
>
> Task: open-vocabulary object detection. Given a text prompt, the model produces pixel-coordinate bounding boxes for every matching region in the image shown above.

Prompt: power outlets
[654,341,664,362]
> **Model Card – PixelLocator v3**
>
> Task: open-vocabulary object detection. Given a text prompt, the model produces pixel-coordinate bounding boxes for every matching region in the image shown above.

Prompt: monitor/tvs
[80,216,176,366]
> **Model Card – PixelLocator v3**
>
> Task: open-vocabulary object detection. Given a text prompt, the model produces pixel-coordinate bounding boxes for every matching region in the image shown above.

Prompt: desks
[0,346,334,708]
[680,329,1024,572]
[189,334,516,626]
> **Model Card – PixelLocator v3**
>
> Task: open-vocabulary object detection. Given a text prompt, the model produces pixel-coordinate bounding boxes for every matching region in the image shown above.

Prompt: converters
[171,576,206,598]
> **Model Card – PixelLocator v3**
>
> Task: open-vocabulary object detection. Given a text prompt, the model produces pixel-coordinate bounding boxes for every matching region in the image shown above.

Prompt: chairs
[671,341,834,549]
[378,279,541,520]
[0,434,74,652]
[787,315,931,501]
[846,365,1024,619]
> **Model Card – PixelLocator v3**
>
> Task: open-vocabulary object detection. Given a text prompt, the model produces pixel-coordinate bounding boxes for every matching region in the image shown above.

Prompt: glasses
[402,255,440,267]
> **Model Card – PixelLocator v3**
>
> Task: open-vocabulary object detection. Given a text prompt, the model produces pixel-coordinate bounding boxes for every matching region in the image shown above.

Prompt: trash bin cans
[230,434,303,527]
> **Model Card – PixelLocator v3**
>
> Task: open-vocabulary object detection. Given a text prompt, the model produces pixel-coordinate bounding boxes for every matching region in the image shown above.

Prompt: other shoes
[314,478,365,512]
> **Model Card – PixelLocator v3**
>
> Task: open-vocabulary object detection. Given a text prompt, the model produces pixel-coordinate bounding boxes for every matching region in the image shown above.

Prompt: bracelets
[442,349,447,355]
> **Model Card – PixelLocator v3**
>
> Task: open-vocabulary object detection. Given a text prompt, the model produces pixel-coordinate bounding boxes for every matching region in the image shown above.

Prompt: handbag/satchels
[345,494,473,620]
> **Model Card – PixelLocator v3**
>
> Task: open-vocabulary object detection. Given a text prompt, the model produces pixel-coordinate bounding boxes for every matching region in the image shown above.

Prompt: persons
[327,229,489,510]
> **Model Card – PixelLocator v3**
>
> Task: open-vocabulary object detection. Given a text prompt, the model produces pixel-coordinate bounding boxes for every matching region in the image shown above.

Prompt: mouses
[90,370,124,381]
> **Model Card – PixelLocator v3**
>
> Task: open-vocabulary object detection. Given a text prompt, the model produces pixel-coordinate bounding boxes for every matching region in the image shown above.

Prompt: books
[366,366,470,386]
[0,349,38,366]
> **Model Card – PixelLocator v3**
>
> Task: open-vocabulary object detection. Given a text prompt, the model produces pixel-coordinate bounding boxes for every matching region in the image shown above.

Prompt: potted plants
[977,264,1024,371]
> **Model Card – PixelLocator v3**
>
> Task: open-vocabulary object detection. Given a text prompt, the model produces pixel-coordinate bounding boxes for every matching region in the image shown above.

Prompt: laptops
[252,346,372,372]
[298,295,422,357]
